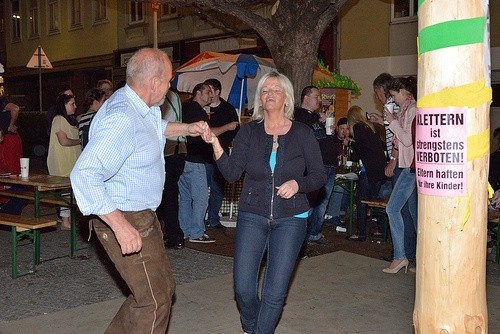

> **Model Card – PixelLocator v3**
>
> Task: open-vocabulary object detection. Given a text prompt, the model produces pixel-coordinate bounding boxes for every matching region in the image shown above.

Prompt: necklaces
[264,115,286,143]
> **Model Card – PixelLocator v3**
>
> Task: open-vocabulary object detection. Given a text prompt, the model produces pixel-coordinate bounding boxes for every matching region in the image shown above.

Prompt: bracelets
[213,148,223,153]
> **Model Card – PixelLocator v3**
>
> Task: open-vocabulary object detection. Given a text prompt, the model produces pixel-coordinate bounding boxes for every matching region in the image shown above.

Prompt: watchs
[389,156,397,160]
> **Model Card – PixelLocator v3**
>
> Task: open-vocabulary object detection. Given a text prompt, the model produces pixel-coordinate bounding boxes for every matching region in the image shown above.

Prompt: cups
[384,104,394,124]
[20,158,30,177]
[325,117,335,135]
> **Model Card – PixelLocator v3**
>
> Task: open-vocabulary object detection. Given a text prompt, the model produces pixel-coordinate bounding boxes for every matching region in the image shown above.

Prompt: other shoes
[57,217,80,230]
[383,251,414,262]
[174,222,226,249]
[307,215,389,246]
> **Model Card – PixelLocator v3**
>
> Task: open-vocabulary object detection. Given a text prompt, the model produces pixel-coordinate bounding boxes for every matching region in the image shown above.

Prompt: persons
[204,78,242,230]
[68,48,212,334]
[44,86,84,223]
[292,85,338,261]
[367,72,418,265]
[486,126,500,259]
[46,93,84,233]
[0,96,24,210]
[176,83,216,244]
[155,88,187,251]
[198,71,328,334]
[96,80,116,102]
[323,117,362,232]
[77,87,108,152]
[341,105,391,243]
[381,74,418,274]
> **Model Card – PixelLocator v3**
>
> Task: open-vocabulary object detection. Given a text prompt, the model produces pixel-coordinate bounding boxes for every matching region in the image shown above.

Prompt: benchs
[361,198,392,244]
[0,213,57,281]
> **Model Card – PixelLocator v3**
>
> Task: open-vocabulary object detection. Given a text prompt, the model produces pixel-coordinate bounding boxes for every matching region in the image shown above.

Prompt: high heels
[382,256,409,274]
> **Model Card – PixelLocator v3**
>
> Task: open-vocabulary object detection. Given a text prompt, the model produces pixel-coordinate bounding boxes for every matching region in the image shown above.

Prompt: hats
[337,118,348,126]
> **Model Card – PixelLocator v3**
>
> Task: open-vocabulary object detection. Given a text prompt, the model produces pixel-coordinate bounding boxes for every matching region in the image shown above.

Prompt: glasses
[260,88,283,95]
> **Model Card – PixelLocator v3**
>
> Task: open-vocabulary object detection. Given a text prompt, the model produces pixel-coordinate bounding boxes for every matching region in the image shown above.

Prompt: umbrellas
[168,48,278,130]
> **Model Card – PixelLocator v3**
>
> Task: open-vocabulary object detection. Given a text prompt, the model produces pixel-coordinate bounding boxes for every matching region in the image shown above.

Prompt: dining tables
[334,165,360,236]
[0,173,77,266]
